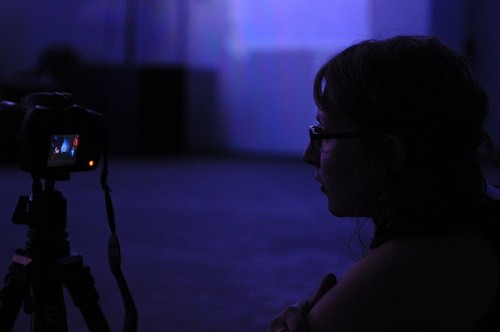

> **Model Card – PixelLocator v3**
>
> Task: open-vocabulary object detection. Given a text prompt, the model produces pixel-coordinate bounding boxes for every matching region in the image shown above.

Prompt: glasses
[308,125,360,149]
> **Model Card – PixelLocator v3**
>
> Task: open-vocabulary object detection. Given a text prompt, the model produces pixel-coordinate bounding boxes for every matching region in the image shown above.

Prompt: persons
[268,30,500,332]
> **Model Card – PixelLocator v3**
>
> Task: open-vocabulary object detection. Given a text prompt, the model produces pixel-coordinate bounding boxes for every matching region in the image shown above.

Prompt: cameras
[0,93,104,182]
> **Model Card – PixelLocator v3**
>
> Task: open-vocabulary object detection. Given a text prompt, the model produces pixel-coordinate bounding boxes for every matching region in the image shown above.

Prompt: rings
[276,327,287,332]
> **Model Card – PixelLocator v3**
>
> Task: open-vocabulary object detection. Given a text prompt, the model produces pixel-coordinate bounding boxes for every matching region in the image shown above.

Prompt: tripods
[1,191,112,332]
